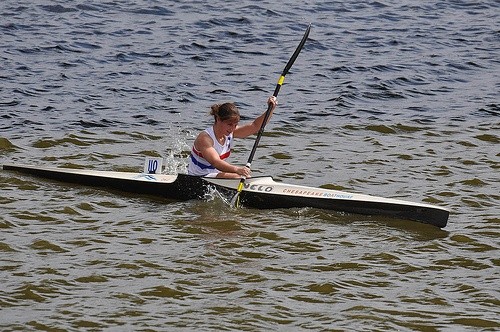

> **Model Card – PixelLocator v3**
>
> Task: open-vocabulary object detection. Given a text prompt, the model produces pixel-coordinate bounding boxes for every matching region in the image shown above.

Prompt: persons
[188,96,278,179]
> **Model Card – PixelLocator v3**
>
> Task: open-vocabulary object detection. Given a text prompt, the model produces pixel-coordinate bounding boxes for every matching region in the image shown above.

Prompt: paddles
[229,22,312,207]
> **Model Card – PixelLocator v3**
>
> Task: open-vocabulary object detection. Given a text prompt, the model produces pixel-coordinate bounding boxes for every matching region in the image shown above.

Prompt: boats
[3,163,452,229]
